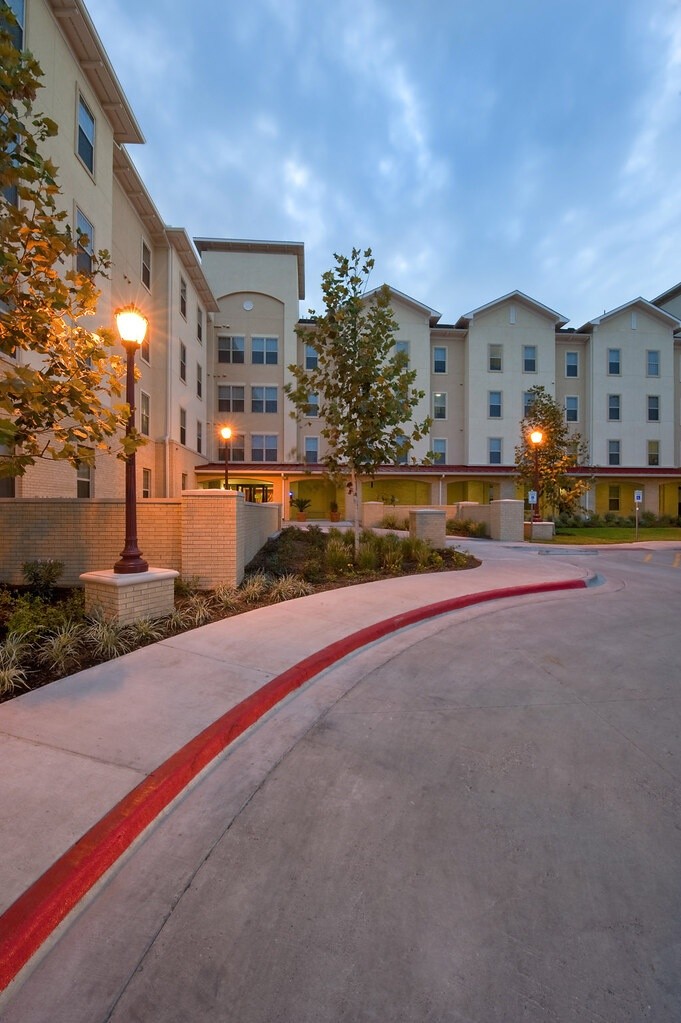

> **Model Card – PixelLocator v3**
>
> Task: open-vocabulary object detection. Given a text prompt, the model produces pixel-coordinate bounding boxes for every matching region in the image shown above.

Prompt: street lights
[530,430,544,522]
[113,304,152,572]
[220,424,232,490]
